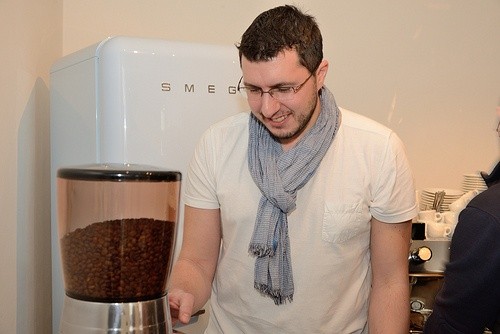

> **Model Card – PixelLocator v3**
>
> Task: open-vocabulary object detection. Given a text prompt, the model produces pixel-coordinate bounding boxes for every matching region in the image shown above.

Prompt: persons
[169,5,418,334]
[424,160,500,334]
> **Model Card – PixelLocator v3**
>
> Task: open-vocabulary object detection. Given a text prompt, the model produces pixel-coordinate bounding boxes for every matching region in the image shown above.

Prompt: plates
[419,186,464,212]
[463,174,489,193]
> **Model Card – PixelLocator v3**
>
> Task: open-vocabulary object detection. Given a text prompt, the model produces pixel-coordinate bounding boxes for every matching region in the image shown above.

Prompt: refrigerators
[48,36,250,334]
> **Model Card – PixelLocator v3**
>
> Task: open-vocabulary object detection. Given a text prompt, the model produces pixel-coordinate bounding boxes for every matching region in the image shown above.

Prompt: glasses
[237,64,319,100]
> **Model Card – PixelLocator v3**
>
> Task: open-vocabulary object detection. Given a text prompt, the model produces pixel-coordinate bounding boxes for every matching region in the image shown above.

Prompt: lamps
[408,246,433,267]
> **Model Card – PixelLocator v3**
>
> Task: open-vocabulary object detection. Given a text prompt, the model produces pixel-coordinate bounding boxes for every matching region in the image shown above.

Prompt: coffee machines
[57,164,182,334]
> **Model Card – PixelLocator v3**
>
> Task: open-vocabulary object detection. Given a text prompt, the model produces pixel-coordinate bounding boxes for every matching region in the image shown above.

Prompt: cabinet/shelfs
[409,240,450,276]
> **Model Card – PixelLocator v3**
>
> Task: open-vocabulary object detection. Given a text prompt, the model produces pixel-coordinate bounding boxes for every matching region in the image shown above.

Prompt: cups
[411,209,456,239]
[449,190,480,214]
